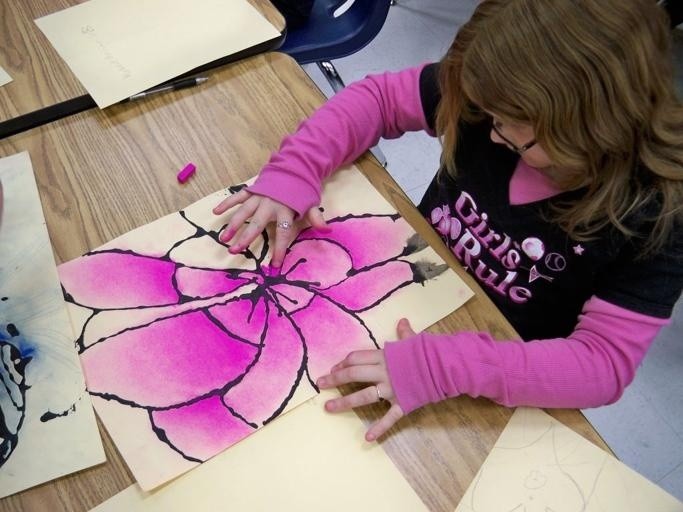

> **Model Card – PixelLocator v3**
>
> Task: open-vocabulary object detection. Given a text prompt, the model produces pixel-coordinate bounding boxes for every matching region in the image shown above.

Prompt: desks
[0,0,619,511]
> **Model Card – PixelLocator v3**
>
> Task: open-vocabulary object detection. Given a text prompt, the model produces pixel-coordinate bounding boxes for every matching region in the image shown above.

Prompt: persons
[212,0,682,441]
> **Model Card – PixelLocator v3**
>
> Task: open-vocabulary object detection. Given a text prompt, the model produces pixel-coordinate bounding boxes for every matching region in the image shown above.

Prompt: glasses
[477,108,538,153]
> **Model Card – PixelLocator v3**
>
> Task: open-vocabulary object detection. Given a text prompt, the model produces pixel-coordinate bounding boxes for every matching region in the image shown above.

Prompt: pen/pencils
[130,76,209,101]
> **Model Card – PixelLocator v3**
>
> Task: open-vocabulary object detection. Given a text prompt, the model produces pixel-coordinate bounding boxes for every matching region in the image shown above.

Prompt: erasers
[177,164,197,182]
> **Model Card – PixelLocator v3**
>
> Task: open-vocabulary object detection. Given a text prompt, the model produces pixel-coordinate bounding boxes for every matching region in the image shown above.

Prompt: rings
[275,221,292,230]
